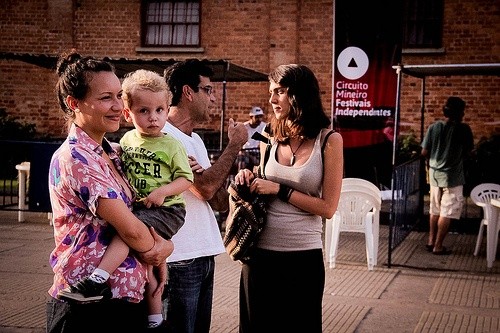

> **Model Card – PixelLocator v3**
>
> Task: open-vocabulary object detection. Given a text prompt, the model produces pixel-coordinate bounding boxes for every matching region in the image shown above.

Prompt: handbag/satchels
[223,122,273,262]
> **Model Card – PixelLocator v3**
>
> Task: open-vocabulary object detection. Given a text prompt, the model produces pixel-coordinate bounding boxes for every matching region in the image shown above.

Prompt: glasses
[197,86,211,95]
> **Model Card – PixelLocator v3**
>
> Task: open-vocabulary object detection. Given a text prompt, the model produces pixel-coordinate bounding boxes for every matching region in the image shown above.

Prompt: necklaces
[288,141,304,165]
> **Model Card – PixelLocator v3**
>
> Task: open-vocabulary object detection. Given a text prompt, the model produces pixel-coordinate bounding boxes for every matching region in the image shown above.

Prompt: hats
[249,107,265,116]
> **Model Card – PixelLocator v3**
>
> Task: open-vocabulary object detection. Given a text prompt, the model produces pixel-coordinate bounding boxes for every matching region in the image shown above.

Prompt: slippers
[432,247,452,255]
[428,246,434,251]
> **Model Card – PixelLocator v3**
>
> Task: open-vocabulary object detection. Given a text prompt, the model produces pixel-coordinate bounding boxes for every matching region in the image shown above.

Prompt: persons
[56,70,194,333]
[161,62,249,333]
[47,50,175,333]
[421,96,474,255]
[234,64,343,333]
[242,107,266,150]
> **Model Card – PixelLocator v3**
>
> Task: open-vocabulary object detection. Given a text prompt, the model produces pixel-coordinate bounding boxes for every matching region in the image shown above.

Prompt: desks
[487,198,500,268]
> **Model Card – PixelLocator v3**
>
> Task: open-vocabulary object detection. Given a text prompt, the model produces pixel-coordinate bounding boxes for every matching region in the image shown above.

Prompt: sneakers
[57,277,112,301]
[144,320,173,333]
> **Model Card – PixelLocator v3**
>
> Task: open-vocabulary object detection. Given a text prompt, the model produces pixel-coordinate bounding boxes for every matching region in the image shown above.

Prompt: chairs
[324,178,381,271]
[470,183,500,256]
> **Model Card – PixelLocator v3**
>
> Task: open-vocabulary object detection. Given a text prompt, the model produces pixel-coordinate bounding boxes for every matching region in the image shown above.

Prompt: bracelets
[277,184,291,201]
[288,188,295,201]
